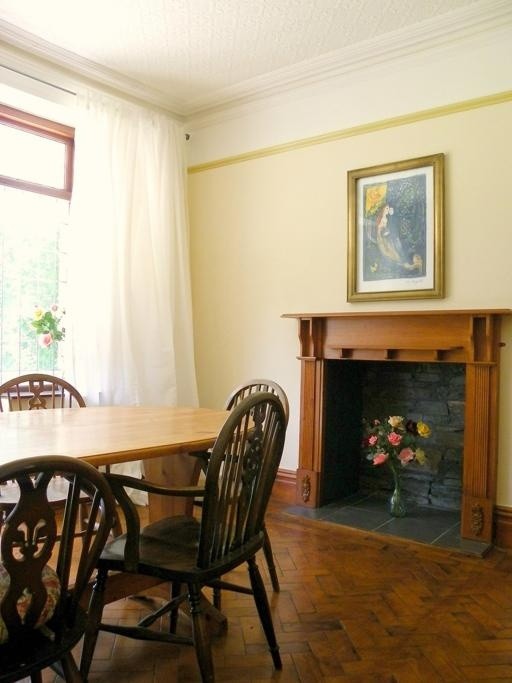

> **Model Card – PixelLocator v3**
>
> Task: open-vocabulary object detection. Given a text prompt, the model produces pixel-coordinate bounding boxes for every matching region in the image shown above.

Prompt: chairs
[0,456,115,683]
[80,392,287,682]
[0,373,122,561]
[193,380,289,591]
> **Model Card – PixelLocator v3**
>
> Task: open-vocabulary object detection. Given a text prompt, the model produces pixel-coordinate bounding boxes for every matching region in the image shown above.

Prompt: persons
[376,203,423,272]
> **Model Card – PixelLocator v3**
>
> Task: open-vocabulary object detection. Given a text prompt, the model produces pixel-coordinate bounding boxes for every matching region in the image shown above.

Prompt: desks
[0,406,255,639]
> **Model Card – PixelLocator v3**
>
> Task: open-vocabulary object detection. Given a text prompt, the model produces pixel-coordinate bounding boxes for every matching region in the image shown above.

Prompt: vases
[389,465,408,518]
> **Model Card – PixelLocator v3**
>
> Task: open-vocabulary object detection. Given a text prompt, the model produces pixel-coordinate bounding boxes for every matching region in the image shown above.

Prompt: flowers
[28,301,69,349]
[363,415,432,470]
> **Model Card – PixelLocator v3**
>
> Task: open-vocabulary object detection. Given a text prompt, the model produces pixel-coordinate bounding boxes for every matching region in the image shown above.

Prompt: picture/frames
[346,154,444,299]
